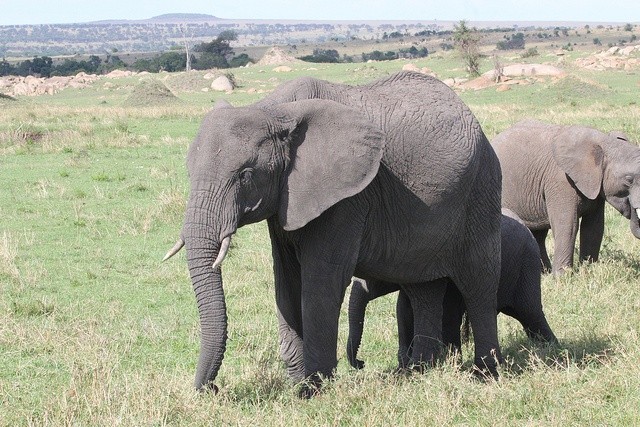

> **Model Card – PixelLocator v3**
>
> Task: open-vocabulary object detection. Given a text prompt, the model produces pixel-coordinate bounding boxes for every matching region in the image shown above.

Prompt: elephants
[160,69,503,400]
[346,207,565,376]
[488,118,640,278]
